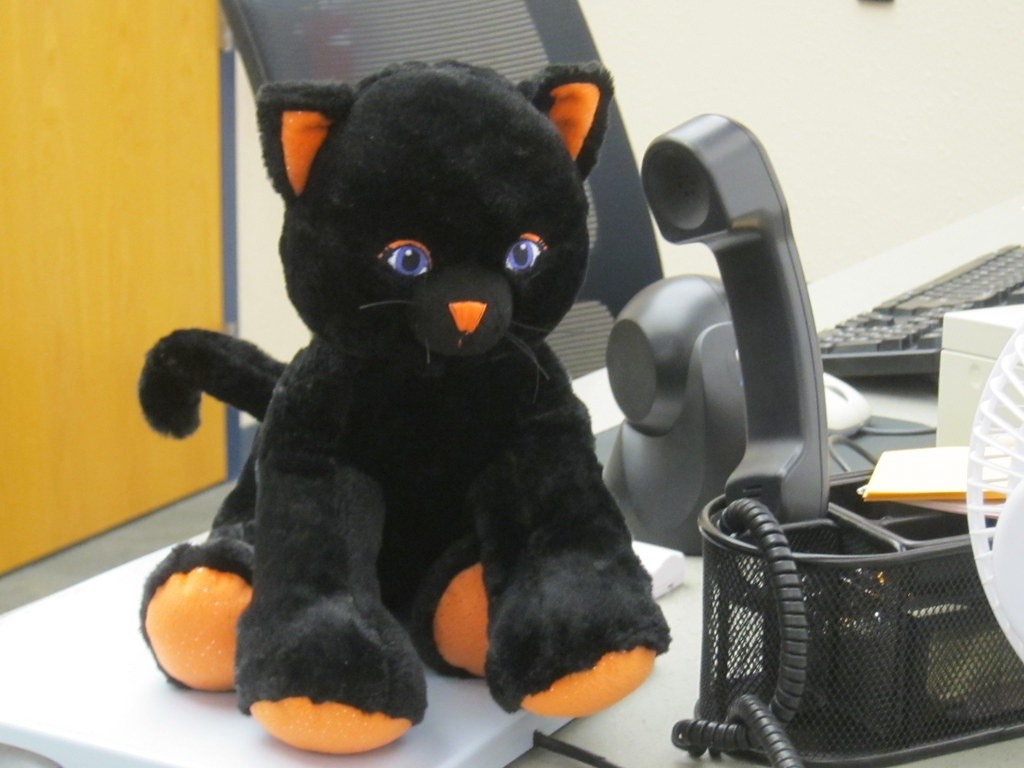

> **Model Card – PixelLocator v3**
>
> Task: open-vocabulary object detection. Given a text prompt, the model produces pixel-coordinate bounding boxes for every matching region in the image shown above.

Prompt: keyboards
[815,245,1024,377]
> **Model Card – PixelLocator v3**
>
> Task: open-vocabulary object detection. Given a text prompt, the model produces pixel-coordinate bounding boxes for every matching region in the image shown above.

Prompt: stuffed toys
[137,58,673,755]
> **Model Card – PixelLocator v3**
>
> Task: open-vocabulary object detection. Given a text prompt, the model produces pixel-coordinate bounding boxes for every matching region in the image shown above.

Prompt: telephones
[637,110,831,553]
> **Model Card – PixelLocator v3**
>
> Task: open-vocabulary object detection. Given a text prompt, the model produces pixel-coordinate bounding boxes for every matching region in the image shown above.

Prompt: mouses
[820,371,870,438]
